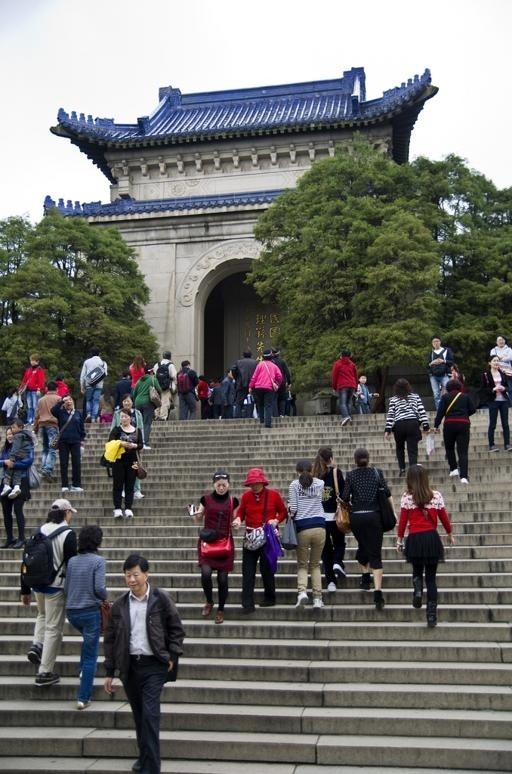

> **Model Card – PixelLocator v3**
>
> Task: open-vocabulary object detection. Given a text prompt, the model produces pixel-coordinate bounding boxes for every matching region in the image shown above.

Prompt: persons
[64,524,110,710]
[80,349,144,517]
[129,347,296,428]
[19,497,77,685]
[384,336,511,483]
[189,446,453,629]
[332,351,379,426]
[1,353,86,548]
[104,552,185,771]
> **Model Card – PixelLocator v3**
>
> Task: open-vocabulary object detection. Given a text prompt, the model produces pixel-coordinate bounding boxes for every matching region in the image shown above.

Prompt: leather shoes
[76,701,91,708]
[202,600,224,623]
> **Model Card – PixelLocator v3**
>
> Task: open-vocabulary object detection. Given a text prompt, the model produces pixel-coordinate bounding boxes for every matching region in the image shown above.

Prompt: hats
[261,349,274,359]
[243,468,269,487]
[50,499,77,513]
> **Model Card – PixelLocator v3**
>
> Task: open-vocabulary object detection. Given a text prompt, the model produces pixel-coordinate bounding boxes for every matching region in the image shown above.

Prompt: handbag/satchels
[136,462,147,479]
[149,385,162,407]
[243,526,267,550]
[377,487,396,532]
[84,365,107,386]
[281,515,298,550]
[271,381,280,393]
[334,497,352,534]
[50,432,61,450]
[101,599,115,636]
[28,465,40,488]
[199,528,232,558]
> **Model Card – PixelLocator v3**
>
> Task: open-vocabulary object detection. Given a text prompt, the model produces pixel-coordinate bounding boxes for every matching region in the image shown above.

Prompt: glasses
[214,475,228,478]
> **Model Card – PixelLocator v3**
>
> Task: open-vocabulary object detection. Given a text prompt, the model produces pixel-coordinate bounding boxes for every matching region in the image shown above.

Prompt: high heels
[0,538,27,549]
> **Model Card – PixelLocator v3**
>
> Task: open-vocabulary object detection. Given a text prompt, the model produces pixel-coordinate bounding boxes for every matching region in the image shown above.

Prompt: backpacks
[155,361,173,391]
[21,526,71,587]
[177,369,192,393]
[431,350,445,377]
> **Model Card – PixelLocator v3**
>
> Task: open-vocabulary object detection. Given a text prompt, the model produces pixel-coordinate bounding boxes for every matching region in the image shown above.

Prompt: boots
[413,576,437,628]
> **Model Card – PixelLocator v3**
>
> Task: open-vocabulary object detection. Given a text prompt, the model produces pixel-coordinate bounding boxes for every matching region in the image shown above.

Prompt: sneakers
[1,484,22,499]
[113,490,145,518]
[449,469,470,485]
[240,599,276,614]
[61,486,84,492]
[341,418,350,426]
[295,563,384,611]
[36,467,57,482]
[487,444,512,452]
[27,645,60,687]
